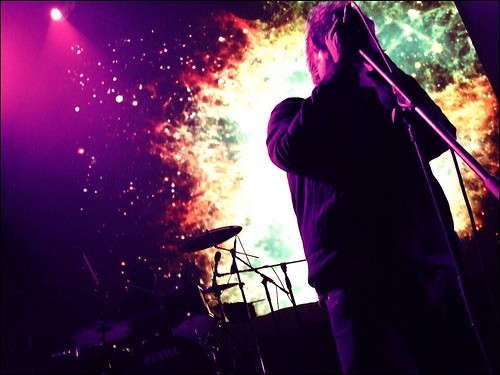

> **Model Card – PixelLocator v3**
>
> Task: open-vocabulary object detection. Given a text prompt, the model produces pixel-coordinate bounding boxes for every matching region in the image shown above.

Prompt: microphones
[342,1,354,27]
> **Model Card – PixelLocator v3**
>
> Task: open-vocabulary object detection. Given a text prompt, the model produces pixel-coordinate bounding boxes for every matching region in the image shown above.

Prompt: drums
[70,302,218,375]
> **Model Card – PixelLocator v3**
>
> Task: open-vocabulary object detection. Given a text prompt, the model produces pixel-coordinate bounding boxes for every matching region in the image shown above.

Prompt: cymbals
[203,283,239,295]
[179,225,242,254]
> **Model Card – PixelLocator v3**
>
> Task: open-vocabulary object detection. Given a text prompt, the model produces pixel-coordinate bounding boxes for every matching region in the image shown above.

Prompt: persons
[265,2,476,375]
